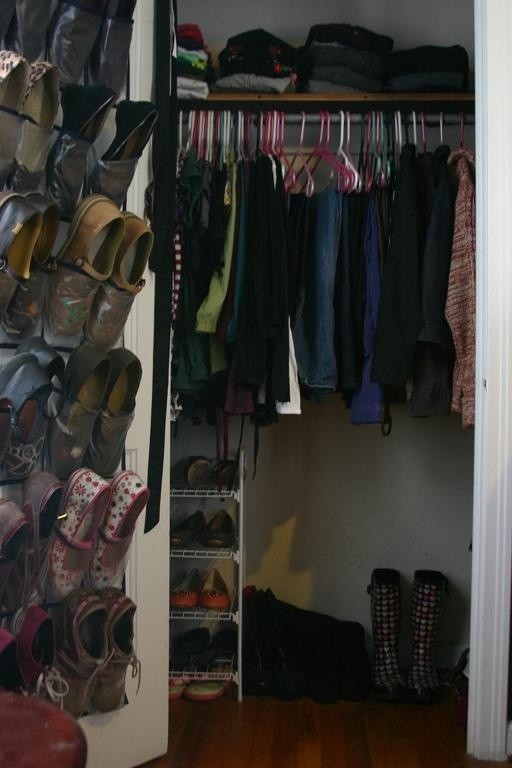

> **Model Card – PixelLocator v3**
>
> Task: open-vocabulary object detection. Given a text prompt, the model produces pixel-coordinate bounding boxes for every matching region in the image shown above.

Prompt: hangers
[174,108,478,199]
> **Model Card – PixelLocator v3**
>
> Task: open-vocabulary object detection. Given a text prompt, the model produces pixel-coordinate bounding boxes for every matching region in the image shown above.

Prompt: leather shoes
[5,2,54,63]
[84,348,142,475]
[172,509,206,546]
[193,625,238,669]
[186,455,213,491]
[211,460,246,492]
[43,194,127,337]
[0,50,30,184]
[204,509,234,547]
[200,569,230,610]
[173,628,209,667]
[9,193,61,334]
[171,568,200,608]
[16,335,66,412]
[0,193,43,328]
[45,2,105,89]
[85,211,153,345]
[45,82,117,219]
[8,61,61,186]
[89,99,161,209]
[1,351,53,480]
[43,345,112,478]
[90,0,137,98]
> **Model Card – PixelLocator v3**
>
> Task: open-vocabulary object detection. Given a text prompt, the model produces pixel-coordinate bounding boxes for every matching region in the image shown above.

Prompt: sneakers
[50,467,112,597]
[55,588,108,717]
[23,469,63,603]
[0,627,28,696]
[1,497,30,611]
[93,588,141,711]
[89,470,150,590]
[14,604,70,706]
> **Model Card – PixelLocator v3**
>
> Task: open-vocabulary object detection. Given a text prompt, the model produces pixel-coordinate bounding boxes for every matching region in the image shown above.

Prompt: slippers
[190,665,232,702]
[169,679,185,699]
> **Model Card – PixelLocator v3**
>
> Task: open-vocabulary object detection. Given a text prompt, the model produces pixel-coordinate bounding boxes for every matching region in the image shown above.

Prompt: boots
[410,569,446,707]
[368,568,408,704]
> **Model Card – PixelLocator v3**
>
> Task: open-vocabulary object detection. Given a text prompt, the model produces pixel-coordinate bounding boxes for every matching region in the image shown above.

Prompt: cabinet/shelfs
[172,447,247,709]
